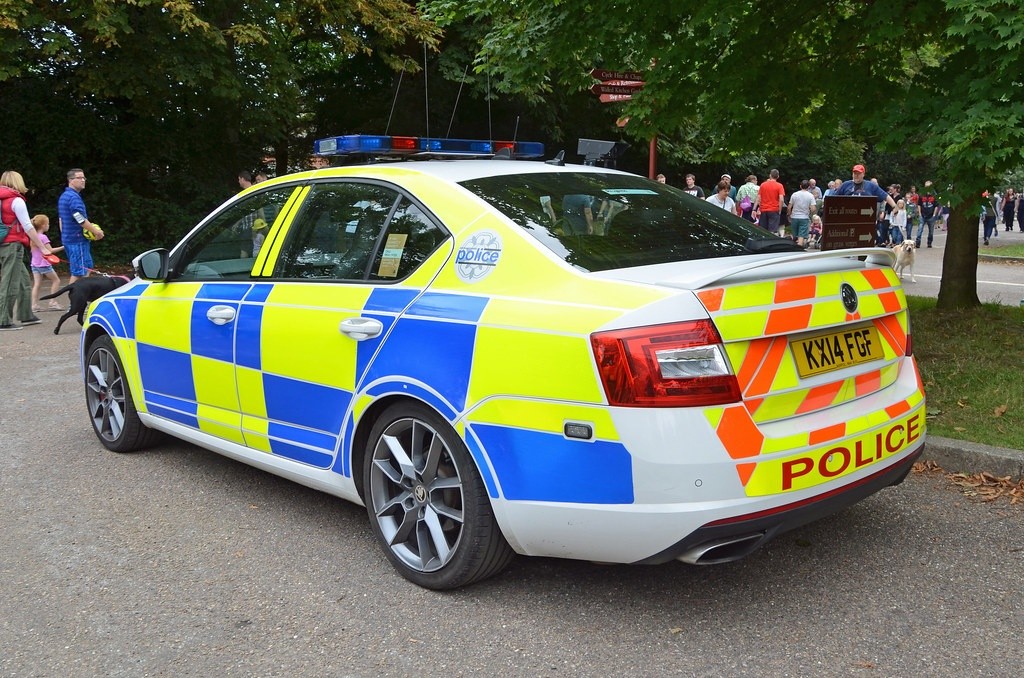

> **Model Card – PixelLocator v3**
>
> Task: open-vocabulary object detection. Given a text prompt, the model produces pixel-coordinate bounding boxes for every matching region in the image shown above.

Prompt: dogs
[39,273,139,335]
[892,240,917,283]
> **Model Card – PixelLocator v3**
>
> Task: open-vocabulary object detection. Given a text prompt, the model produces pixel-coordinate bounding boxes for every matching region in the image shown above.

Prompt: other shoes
[916,241,921,247]
[32,305,46,312]
[927,244,932,248]
[995,230,998,235]
[0,324,23,331]
[49,302,65,310]
[22,316,42,325]
[984,240,989,245]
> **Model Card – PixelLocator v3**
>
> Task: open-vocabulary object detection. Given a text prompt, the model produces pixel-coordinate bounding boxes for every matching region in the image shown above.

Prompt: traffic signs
[821,196,878,251]
[588,66,648,103]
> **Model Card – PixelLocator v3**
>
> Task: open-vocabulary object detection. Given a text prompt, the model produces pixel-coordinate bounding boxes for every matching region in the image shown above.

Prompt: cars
[80,134,927,591]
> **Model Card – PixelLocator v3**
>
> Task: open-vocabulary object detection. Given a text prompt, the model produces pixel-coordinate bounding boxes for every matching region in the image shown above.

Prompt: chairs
[607,209,643,237]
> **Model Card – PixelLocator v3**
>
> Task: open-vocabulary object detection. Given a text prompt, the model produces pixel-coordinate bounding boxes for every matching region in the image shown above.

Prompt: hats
[722,174,731,180]
[853,165,864,173]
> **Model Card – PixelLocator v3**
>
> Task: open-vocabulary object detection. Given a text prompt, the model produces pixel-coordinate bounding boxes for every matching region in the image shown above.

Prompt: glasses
[71,177,86,180]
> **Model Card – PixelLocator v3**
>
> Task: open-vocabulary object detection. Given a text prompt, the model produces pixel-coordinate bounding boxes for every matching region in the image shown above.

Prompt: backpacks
[740,185,754,211]
[0,201,18,242]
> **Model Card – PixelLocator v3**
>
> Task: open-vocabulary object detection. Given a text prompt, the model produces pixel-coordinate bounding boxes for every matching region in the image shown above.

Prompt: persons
[706,181,738,216]
[712,173,737,205]
[230,170,280,260]
[981,187,1024,245]
[750,169,785,233]
[777,202,788,237]
[807,176,842,244]
[870,177,950,248]
[29,214,67,311]
[537,192,628,236]
[682,173,705,200]
[0,171,53,330]
[786,179,816,245]
[657,174,666,184]
[833,164,898,217]
[57,169,104,285]
[734,174,760,222]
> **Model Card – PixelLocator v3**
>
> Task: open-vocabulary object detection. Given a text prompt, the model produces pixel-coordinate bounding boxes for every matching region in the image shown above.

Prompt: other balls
[82,223,101,241]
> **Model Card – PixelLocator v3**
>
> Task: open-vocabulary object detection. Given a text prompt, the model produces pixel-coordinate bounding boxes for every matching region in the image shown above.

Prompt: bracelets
[892,207,895,210]
